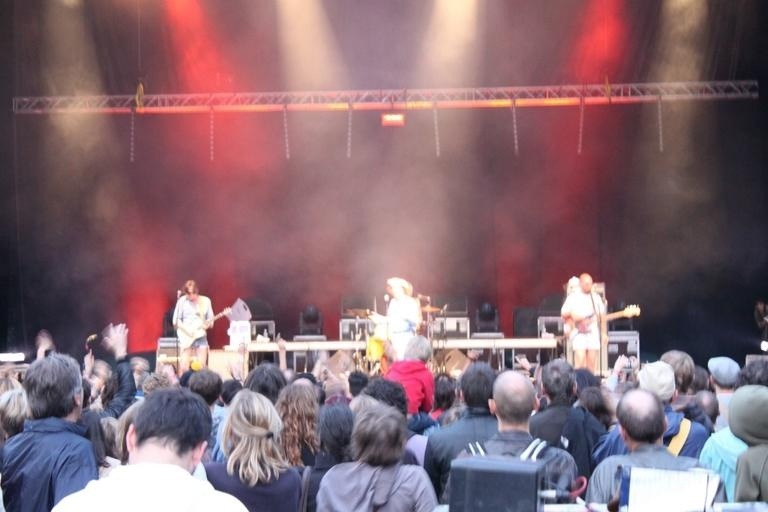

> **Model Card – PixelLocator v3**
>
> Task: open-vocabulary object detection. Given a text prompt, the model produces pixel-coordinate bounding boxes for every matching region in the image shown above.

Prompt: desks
[246,337,561,379]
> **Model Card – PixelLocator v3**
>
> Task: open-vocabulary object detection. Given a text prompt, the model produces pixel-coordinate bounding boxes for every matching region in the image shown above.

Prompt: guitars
[176,307,232,347]
[564,305,640,338]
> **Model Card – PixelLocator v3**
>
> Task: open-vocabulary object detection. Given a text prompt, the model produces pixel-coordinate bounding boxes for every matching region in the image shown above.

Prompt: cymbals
[423,306,440,313]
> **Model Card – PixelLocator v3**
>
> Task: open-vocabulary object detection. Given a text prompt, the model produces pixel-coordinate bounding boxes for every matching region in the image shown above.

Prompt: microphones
[594,282,600,294]
[384,294,389,311]
[177,290,182,299]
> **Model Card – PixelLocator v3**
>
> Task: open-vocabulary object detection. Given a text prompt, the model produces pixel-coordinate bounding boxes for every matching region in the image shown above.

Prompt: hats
[707,355,743,388]
